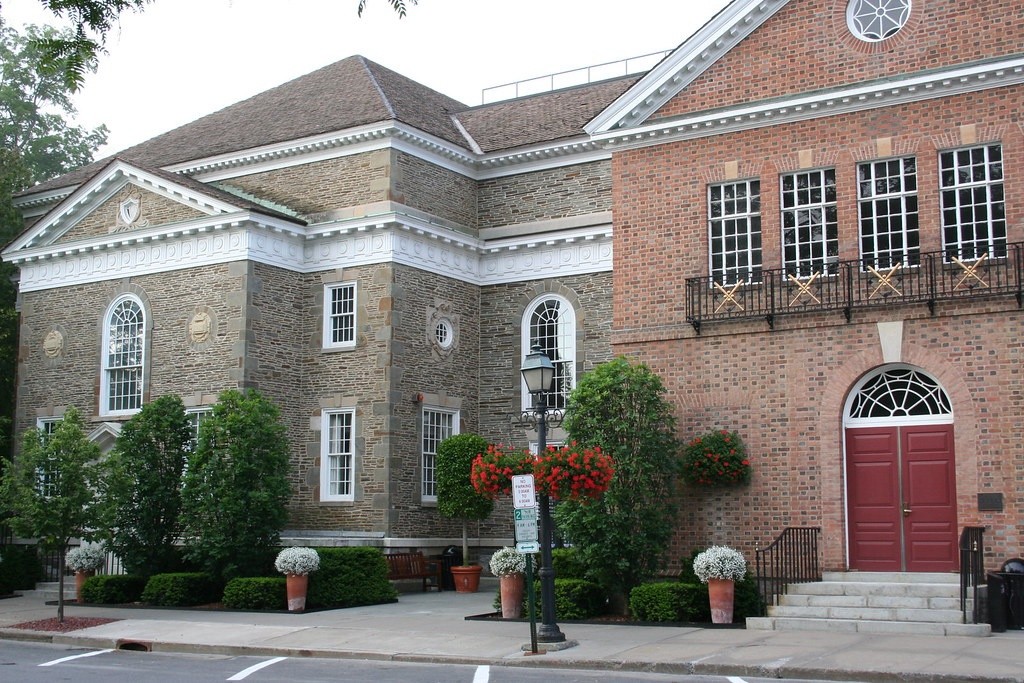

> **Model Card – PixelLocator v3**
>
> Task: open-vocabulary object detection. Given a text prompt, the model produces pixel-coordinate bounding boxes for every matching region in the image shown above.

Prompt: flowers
[679,429,751,491]
[273,545,320,578]
[470,442,616,509]
[692,544,747,585]
[64,544,106,574]
[489,546,538,580]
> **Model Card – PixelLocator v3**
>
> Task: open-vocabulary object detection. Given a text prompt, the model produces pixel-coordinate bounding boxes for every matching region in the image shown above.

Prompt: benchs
[383,551,443,593]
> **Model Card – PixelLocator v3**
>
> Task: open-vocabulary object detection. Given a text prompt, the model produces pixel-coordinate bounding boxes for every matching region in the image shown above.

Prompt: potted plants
[434,434,496,593]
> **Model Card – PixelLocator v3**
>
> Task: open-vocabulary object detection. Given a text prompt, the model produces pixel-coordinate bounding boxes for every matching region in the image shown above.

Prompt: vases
[501,573,525,619]
[287,573,308,612]
[75,570,95,603]
[708,577,736,624]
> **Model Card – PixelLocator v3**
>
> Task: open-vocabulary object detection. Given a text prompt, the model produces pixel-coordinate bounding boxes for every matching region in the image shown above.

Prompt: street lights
[506,338,579,643]
